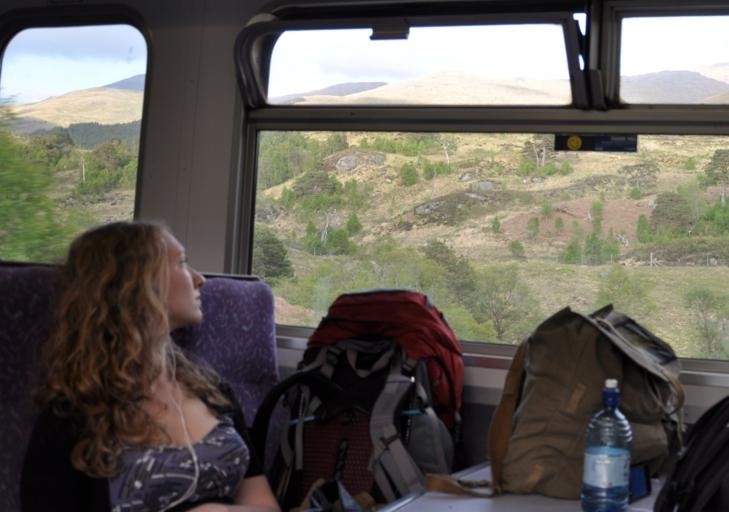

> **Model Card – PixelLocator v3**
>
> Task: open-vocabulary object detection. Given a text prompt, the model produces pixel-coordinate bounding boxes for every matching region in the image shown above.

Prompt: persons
[15,217,284,511]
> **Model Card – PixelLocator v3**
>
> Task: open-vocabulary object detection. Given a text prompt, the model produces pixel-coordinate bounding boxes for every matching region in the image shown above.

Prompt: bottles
[579,378,634,512]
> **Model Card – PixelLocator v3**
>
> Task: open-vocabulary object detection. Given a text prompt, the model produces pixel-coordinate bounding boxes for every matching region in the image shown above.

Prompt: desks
[370,424,692,512]
